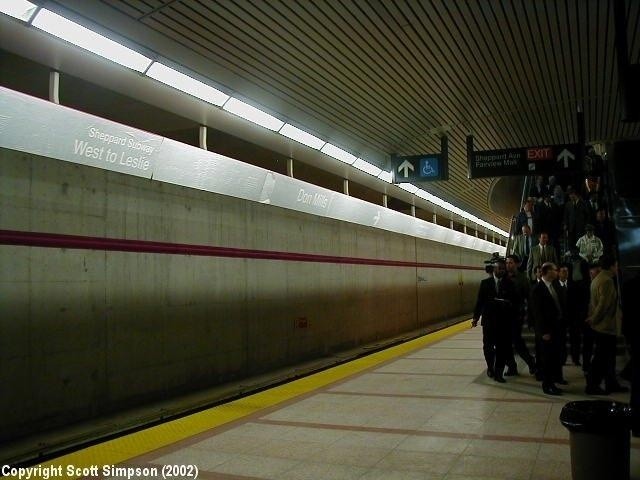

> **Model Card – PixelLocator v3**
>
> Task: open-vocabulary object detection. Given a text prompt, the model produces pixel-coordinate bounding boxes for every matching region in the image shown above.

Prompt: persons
[470,171,634,395]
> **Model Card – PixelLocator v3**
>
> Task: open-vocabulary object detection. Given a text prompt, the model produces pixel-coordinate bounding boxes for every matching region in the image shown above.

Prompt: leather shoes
[486,366,518,384]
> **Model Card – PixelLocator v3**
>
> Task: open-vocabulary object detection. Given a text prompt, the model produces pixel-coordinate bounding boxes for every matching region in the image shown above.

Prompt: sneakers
[529,367,568,395]
[574,359,629,396]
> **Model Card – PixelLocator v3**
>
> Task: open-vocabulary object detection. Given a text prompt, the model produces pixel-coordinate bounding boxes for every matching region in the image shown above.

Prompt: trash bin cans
[559,399,635,480]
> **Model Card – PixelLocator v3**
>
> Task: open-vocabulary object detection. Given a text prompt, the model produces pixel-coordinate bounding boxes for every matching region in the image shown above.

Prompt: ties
[549,284,562,317]
[525,235,530,257]
[541,245,547,263]
[573,200,577,208]
